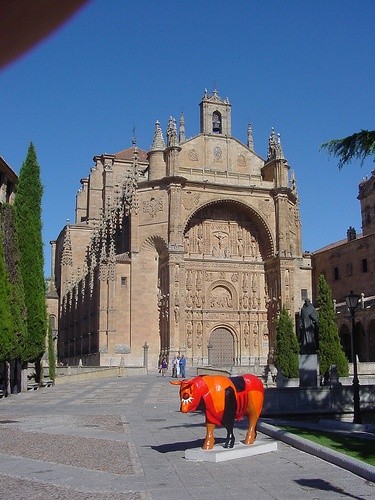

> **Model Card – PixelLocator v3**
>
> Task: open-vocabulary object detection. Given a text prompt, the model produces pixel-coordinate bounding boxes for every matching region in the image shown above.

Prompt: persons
[172,356,178,378]
[159,357,167,378]
[298,298,319,354]
[179,355,186,378]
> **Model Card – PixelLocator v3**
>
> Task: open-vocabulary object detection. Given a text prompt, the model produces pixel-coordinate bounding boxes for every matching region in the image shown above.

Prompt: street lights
[343,290,363,424]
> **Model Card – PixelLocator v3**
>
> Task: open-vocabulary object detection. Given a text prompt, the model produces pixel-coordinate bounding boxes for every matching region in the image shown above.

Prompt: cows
[170,374,264,449]
